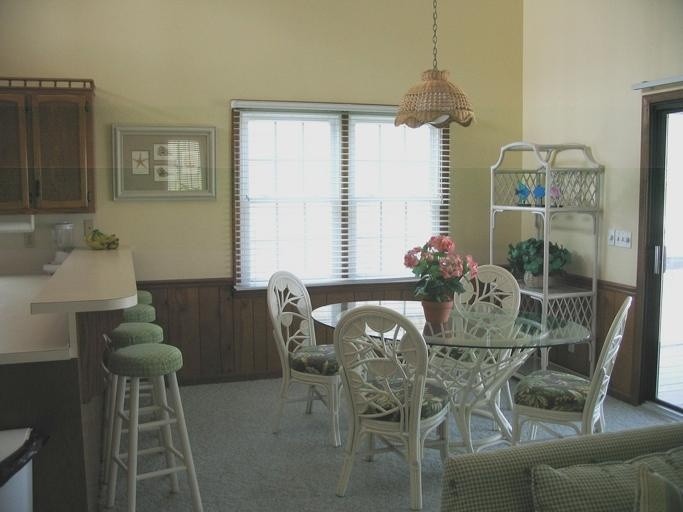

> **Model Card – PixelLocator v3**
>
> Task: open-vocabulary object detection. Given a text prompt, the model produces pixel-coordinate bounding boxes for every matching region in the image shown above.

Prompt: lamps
[392,0,475,131]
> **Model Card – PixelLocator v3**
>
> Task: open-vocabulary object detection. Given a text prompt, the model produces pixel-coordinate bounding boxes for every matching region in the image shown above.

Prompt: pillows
[534,448,682,509]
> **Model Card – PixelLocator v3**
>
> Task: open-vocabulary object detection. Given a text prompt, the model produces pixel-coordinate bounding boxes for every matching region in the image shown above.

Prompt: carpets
[101,353,681,511]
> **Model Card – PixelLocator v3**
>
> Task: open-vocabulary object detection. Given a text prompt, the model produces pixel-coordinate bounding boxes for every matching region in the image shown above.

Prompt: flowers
[404,234,481,300]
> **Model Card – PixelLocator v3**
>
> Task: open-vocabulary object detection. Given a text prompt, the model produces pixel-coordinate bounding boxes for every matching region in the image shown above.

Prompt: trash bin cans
[0,427,35,511]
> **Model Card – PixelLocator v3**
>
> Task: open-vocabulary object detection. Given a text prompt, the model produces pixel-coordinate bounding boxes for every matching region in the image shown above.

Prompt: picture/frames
[109,123,217,203]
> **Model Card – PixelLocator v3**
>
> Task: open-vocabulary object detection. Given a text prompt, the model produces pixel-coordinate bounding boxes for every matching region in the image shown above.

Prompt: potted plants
[508,238,571,290]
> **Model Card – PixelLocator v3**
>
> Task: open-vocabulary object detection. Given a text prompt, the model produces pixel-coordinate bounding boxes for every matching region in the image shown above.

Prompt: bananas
[86,229,120,250]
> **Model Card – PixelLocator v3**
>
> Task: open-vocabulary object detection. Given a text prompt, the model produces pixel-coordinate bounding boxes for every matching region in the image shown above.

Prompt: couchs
[438,418,681,510]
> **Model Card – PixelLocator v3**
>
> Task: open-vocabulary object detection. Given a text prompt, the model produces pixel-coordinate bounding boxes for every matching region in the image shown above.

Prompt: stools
[95,287,206,512]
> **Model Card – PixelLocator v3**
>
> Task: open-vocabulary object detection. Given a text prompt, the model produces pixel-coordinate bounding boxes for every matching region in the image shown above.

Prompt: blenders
[44,221,75,273]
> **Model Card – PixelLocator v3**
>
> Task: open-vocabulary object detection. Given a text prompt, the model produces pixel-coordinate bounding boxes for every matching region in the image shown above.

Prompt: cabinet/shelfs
[486,136,605,392]
[0,77,95,215]
